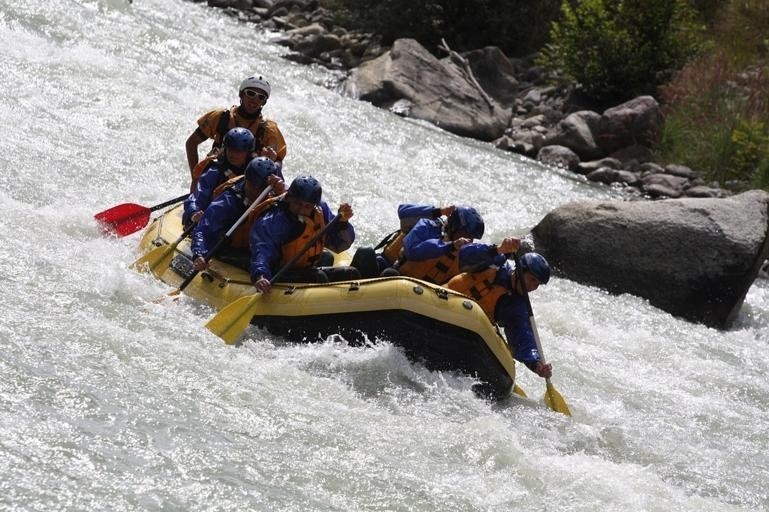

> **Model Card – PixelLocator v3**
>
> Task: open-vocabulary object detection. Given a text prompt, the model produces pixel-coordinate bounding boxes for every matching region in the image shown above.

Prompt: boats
[138,199,516,403]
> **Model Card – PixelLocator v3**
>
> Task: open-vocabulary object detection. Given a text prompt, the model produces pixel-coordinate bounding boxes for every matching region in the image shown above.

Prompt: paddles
[126,224,196,281]
[513,251,572,418]
[204,213,343,345]
[94,194,190,237]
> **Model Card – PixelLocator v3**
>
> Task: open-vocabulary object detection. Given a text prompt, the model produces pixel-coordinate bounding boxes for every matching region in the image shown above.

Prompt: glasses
[243,89,267,101]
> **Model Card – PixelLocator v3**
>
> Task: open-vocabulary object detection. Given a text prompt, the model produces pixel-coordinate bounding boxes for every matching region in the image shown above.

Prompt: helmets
[289,175,322,201]
[436,204,483,241]
[518,252,550,284]
[245,156,278,186]
[239,75,271,98]
[223,127,255,151]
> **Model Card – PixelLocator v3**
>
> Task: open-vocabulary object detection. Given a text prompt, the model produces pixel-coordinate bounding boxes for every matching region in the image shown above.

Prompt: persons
[189,156,289,275]
[375,206,484,282]
[181,127,278,235]
[439,236,553,382]
[184,73,287,173]
[246,172,356,294]
[347,202,456,279]
